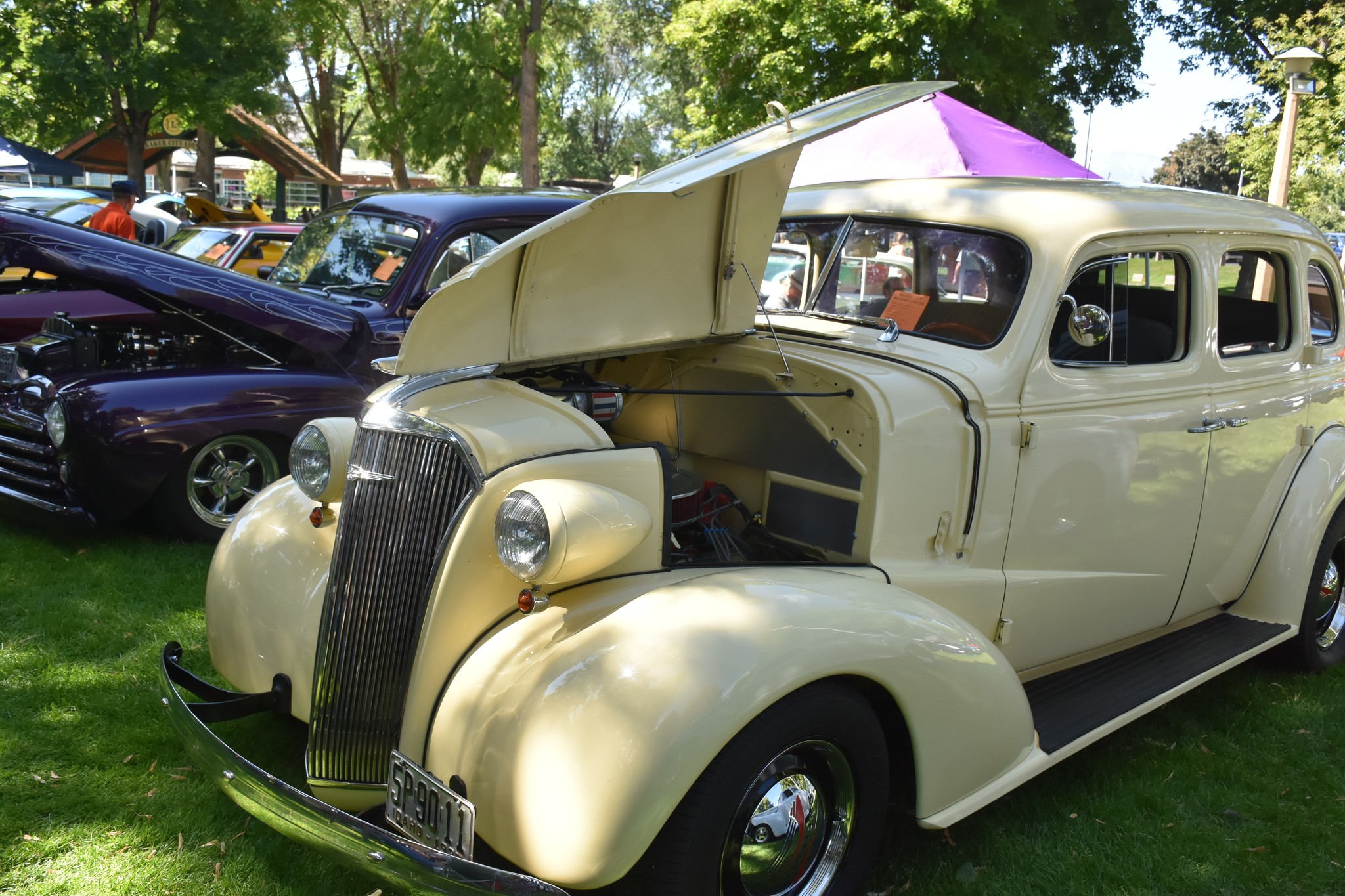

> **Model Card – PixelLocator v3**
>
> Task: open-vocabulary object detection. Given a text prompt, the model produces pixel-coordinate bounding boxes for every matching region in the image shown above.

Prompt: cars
[0,189,596,546]
[0,182,389,296]
[0,220,472,345]
[158,80,1345,896]
[755,242,986,313]
[1323,233,1345,262]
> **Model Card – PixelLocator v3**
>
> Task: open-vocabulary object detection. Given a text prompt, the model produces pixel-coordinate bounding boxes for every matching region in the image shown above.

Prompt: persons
[176,206,189,222]
[763,232,982,309]
[271,208,320,223]
[88,180,142,240]
[226,195,263,211]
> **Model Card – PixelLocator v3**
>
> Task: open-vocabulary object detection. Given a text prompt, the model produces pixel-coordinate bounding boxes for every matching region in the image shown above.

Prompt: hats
[788,267,805,293]
[112,180,142,198]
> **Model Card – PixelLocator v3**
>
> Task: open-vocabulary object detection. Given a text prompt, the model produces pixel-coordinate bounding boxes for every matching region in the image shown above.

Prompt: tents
[789,89,1108,187]
[0,137,87,190]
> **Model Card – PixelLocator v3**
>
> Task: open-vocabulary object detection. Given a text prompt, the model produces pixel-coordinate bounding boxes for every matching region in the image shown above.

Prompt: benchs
[1080,282,1278,342]
[854,291,1172,363]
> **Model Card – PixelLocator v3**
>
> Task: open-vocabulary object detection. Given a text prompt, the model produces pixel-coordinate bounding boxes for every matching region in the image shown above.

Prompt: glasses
[889,287,906,292]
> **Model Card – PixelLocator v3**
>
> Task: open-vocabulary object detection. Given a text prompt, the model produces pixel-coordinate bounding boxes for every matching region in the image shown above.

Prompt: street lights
[1252,45,1323,301]
